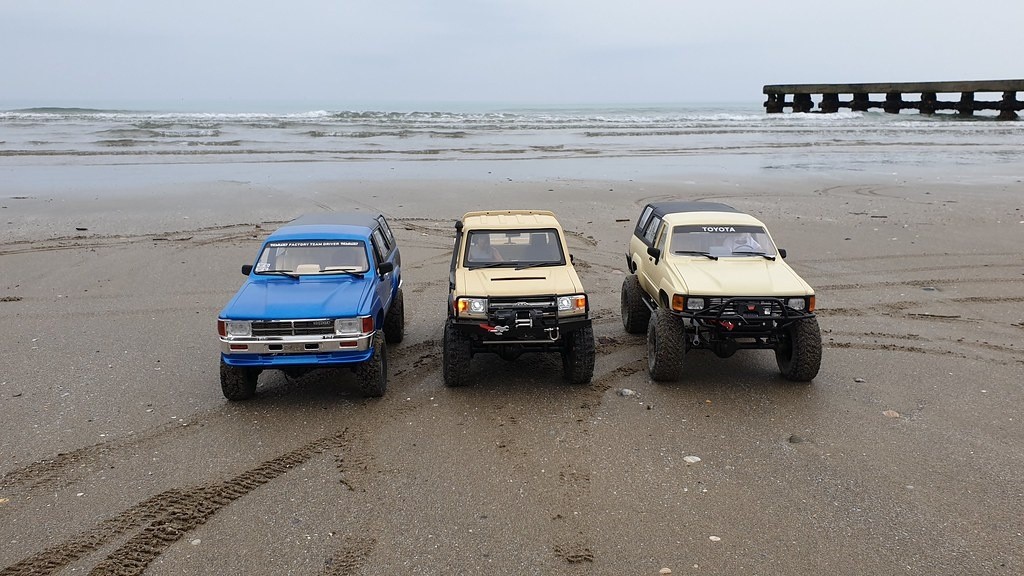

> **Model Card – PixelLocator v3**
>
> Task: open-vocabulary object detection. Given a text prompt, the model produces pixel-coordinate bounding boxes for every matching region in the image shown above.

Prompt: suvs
[441,209,596,387]
[620,201,824,384]
[216,210,405,402]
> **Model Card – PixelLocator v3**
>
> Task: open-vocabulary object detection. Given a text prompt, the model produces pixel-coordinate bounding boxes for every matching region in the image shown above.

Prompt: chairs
[278,249,304,272]
[674,233,701,251]
[527,235,550,259]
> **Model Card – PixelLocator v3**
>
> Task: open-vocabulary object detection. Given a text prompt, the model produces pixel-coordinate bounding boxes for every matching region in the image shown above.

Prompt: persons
[470,234,503,260]
[724,232,764,252]
